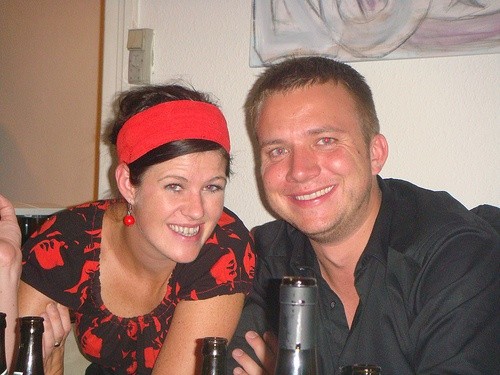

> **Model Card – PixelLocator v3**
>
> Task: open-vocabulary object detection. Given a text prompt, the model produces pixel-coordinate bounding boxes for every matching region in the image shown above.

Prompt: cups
[18,218,37,245]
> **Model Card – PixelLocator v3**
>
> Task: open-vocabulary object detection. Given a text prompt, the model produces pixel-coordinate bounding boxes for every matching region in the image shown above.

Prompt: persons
[227,56,500,375]
[0,84,258,375]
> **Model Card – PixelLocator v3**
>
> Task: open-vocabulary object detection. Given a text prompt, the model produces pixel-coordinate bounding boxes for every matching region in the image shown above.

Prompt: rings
[54,342,60,347]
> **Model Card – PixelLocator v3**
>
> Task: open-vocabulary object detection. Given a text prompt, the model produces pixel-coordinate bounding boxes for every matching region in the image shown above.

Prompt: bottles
[343,364,382,375]
[0,312,8,375]
[274,276,319,375]
[201,337,228,375]
[13,317,44,375]
